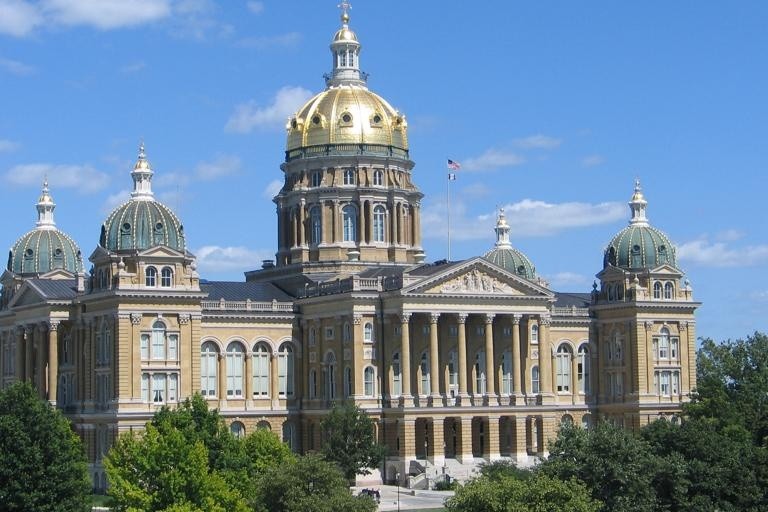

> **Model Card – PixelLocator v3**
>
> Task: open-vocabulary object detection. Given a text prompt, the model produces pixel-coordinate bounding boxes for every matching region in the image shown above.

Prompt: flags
[448,173,455,180]
[447,158,461,170]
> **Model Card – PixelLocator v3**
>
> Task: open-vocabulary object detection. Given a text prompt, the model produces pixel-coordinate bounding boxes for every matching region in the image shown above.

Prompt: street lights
[395,471,401,510]
